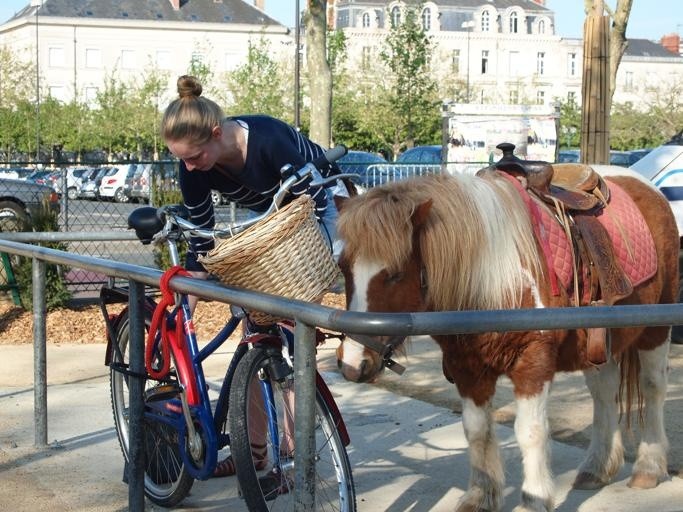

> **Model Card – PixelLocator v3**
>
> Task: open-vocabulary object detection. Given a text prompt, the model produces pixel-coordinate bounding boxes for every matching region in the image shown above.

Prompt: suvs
[0,177,61,247]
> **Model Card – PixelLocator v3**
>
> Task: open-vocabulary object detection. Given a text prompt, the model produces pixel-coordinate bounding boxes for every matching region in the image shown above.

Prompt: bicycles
[98,148,369,512]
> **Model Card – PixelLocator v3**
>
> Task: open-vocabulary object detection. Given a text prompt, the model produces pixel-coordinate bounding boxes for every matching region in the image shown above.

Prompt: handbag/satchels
[122,414,189,486]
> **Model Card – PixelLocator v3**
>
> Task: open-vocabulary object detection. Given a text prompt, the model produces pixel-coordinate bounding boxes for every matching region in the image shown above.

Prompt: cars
[0,158,185,205]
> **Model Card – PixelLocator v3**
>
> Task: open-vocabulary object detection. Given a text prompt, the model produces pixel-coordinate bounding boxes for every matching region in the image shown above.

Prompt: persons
[161,76,358,500]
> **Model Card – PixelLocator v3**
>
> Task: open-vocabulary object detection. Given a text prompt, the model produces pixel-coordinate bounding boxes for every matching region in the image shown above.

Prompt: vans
[625,118,683,350]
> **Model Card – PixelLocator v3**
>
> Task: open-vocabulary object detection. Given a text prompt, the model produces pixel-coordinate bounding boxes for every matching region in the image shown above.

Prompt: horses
[332,164,680,512]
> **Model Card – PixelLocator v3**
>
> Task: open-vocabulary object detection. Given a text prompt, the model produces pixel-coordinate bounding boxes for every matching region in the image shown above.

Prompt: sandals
[258,471,295,502]
[200,444,270,477]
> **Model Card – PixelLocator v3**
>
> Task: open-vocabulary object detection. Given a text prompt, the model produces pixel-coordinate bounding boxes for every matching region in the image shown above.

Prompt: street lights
[459,19,476,104]
[149,90,160,162]
